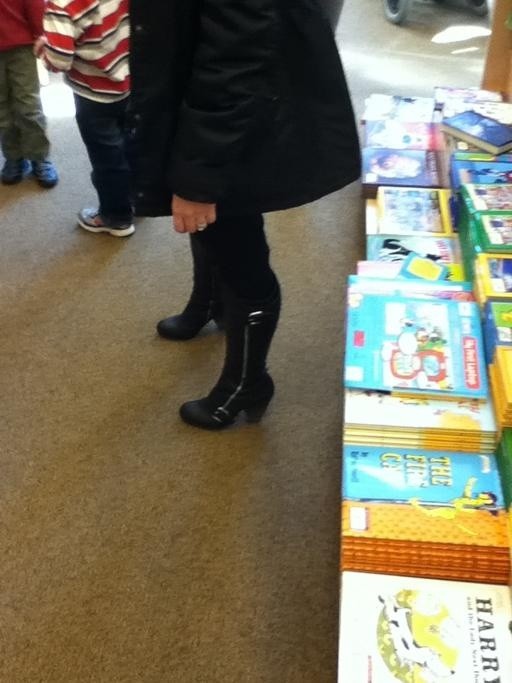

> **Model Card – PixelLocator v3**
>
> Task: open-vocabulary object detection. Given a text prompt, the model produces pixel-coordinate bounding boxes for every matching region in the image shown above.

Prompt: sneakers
[32,161,58,189]
[0,158,29,185]
[76,208,135,238]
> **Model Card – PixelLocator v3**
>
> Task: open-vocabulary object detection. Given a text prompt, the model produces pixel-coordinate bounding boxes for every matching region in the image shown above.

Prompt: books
[338,86,512,683]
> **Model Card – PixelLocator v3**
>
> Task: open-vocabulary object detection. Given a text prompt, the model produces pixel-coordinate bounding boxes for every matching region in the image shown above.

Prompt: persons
[0,0,60,190]
[126,0,354,430]
[30,0,141,238]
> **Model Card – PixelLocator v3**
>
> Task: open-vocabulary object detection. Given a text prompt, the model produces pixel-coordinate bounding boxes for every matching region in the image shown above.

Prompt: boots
[178,285,282,431]
[158,233,229,341]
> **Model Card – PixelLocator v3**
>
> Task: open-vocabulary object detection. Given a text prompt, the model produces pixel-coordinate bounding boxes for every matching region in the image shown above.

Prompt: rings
[197,222,208,232]
